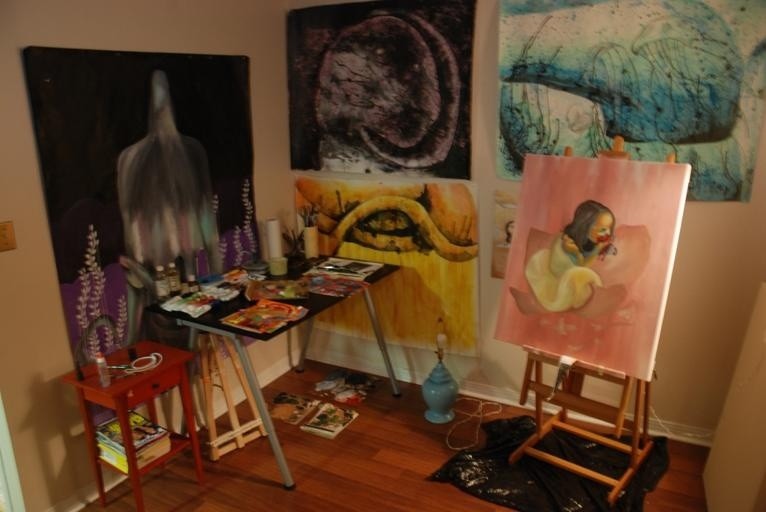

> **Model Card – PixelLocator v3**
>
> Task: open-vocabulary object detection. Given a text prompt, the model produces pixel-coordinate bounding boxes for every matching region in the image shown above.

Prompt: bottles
[153,262,200,303]
[96,352,111,388]
[73,361,84,381]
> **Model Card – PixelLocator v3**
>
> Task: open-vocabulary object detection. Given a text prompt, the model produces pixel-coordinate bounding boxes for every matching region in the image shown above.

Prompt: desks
[144,251,401,490]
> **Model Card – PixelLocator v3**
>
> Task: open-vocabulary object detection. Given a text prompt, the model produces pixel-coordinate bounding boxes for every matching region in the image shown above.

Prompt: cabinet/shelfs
[507,343,655,506]
[63,339,203,512]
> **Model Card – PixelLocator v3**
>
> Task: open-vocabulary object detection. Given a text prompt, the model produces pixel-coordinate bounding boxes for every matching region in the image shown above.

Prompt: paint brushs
[284,231,308,261]
[299,203,322,226]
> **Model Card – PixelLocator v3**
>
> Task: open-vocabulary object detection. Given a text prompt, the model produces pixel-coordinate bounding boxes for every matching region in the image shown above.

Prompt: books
[97,412,171,473]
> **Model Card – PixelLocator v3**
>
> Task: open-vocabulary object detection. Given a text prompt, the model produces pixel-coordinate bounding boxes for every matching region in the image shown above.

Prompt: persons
[526,199,615,312]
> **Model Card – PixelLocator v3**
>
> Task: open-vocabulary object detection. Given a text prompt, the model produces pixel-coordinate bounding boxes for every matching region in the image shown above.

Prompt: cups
[269,257,288,276]
[128,348,137,361]
